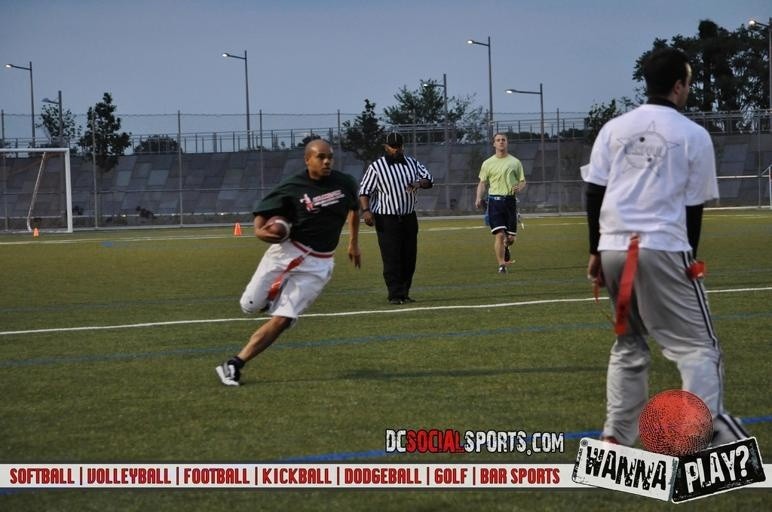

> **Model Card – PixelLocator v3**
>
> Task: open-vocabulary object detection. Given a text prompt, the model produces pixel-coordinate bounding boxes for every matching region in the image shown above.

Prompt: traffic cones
[233,223,242,237]
[33,227,38,237]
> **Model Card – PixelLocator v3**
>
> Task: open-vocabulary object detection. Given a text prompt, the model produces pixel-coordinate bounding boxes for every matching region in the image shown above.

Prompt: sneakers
[215,362,244,388]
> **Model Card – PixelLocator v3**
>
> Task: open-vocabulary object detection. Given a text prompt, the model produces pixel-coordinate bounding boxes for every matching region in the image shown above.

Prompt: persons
[356,131,436,306]
[579,47,751,452]
[476,131,527,277]
[214,138,363,388]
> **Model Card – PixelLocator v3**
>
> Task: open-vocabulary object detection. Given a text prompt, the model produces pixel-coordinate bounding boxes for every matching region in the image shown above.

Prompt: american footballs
[266,217,290,243]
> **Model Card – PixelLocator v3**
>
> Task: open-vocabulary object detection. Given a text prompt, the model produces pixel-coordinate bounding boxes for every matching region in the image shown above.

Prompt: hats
[382,131,403,153]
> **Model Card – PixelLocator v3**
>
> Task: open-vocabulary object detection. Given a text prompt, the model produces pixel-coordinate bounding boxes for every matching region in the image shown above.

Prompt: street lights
[745,19,771,113]
[465,36,495,160]
[504,82,547,208]
[5,61,36,148]
[41,89,63,148]
[220,50,252,151]
[420,73,451,209]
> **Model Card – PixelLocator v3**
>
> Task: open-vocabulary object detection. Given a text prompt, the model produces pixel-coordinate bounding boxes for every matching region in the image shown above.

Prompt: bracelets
[361,207,370,213]
